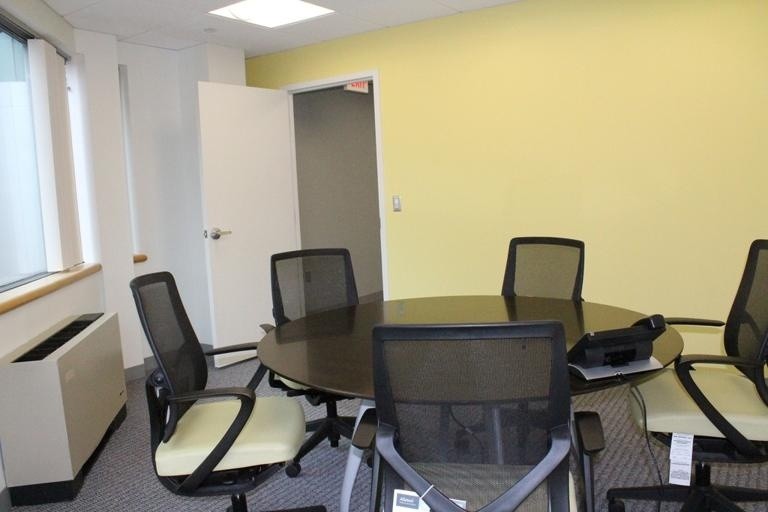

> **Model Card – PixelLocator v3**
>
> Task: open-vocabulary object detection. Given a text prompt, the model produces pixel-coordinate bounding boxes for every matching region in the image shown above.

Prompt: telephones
[567,314,666,383]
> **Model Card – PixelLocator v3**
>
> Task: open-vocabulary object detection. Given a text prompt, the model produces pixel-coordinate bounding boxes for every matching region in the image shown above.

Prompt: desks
[256,294,685,512]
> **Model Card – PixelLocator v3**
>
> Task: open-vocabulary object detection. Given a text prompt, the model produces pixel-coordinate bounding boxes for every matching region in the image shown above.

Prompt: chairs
[605,237,767,512]
[455,236,586,463]
[270,246,399,476]
[126,271,330,512]
[367,316,578,512]
